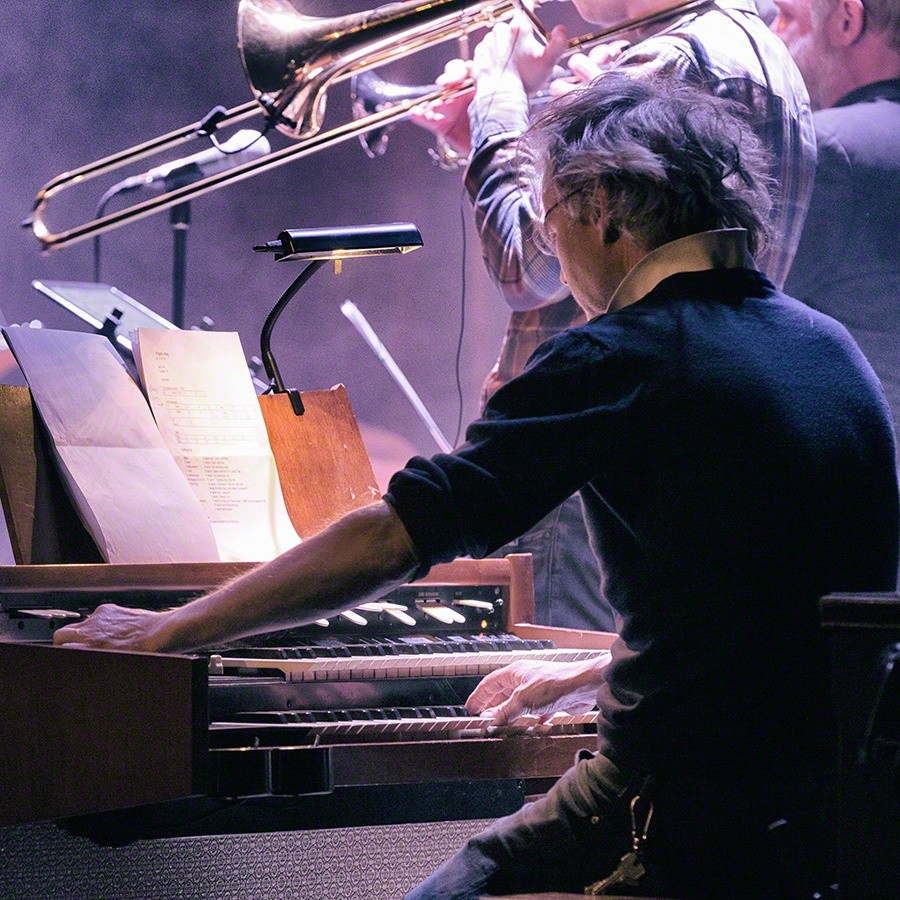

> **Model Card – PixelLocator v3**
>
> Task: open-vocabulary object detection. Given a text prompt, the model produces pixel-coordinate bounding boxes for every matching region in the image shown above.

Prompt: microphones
[120,129,273,196]
[200,105,271,155]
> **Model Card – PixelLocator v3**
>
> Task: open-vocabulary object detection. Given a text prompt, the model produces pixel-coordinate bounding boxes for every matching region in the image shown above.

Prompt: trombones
[29,0,717,248]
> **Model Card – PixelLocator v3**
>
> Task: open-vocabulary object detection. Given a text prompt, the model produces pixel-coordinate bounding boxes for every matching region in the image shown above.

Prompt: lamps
[253,220,425,416]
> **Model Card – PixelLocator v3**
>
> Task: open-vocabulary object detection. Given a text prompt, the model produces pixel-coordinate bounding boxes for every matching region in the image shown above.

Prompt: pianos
[0,550,626,844]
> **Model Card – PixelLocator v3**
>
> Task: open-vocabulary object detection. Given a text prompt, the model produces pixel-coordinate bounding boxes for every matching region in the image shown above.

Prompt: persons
[548,0,900,490]
[52,65,900,899]
[409,1,818,633]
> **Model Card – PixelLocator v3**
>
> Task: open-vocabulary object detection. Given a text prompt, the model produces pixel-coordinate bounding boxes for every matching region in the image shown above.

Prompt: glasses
[532,178,596,259]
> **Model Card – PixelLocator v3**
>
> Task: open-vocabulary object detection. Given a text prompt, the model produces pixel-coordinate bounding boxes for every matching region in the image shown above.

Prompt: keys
[583,853,647,896]
